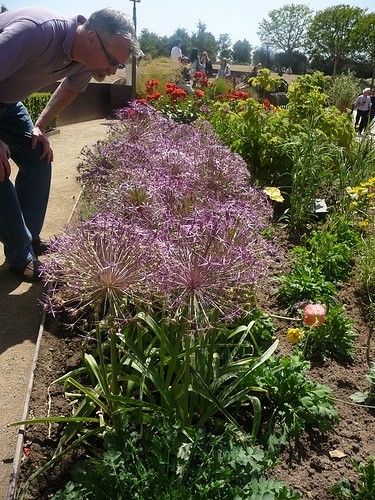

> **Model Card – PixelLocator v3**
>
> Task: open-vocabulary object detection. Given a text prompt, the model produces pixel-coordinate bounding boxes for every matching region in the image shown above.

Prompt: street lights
[129,0,141,105]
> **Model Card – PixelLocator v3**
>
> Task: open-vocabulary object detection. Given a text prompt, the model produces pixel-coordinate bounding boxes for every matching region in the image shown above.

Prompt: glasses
[94,30,126,69]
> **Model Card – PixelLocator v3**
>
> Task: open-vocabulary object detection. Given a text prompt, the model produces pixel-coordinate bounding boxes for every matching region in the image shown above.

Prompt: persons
[189,48,203,74]
[354,89,372,135]
[369,88,375,122]
[0,6,145,283]
[125,48,144,85]
[244,65,314,95]
[171,38,184,67]
[200,52,213,78]
[175,56,196,94]
[215,58,231,80]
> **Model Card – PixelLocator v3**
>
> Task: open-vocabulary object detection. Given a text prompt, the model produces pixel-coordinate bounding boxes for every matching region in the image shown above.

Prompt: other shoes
[33,240,58,255]
[9,257,46,282]
